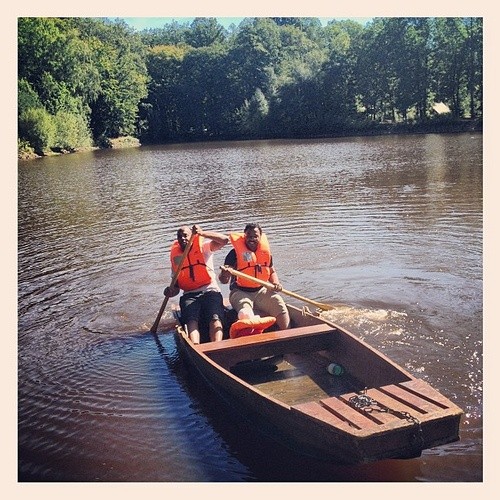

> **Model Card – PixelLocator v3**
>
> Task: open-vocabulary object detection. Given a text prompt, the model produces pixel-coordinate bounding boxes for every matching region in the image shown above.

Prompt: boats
[173,293,464,464]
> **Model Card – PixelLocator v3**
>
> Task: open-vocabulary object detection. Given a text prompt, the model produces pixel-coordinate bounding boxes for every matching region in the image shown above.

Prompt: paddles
[147,232,197,332]
[219,264,330,309]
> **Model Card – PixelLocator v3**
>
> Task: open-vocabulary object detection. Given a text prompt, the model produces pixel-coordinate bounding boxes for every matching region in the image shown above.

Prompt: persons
[164,226,229,344]
[218,222,291,330]
